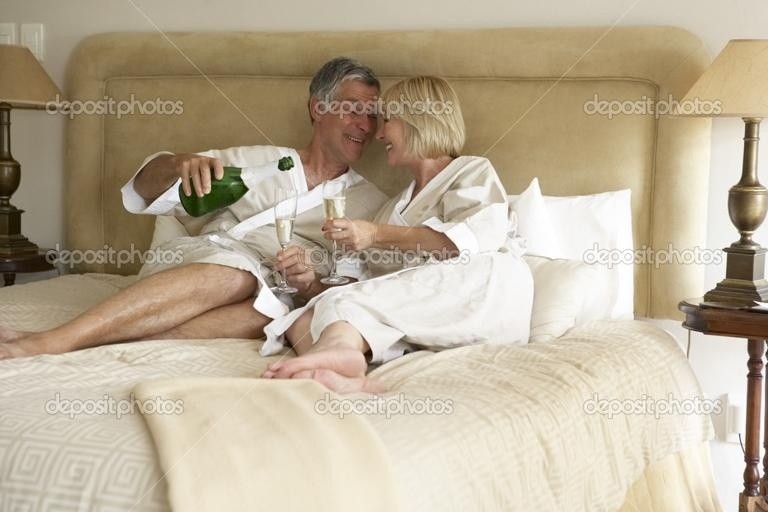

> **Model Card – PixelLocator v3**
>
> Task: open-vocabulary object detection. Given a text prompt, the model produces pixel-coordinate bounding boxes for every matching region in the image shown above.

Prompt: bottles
[177,156,295,218]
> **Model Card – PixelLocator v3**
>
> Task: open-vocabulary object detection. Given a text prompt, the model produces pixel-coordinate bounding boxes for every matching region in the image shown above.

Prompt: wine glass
[320,180,349,287]
[271,187,298,295]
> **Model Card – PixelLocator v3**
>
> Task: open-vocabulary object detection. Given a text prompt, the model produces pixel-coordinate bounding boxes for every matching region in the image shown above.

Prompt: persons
[1,55,392,364]
[260,77,535,397]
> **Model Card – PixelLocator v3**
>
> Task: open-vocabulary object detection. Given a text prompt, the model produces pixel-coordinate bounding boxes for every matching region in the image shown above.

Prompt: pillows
[507,180,557,264]
[507,177,609,346]
[521,254,611,343]
[133,216,189,281]
[512,188,642,325]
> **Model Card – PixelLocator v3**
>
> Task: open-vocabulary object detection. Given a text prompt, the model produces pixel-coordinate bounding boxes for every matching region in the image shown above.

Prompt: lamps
[0,44,69,261]
[672,39,768,309]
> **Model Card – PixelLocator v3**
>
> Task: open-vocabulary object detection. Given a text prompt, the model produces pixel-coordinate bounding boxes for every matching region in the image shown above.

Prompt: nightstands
[0,250,55,287]
[677,296,768,512]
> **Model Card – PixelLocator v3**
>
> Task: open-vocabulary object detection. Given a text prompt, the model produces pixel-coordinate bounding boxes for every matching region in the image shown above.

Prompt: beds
[0,28,709,512]
